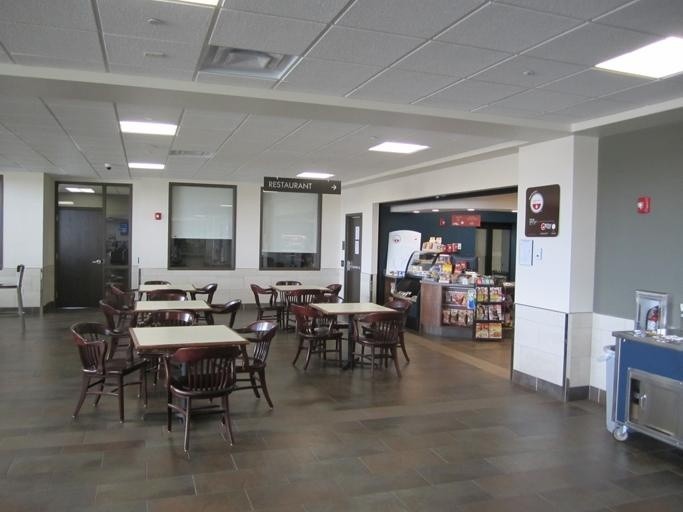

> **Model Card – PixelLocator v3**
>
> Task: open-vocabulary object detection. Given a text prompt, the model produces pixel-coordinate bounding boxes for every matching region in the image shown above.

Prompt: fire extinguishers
[645,305,659,331]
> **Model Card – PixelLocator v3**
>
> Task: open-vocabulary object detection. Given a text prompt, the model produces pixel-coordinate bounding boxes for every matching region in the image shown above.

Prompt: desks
[128,326,249,426]
[308,301,398,372]
[611,328,682,449]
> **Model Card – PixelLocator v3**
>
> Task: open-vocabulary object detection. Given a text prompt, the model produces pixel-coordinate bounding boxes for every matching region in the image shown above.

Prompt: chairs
[70,321,150,422]
[305,303,341,361]
[212,322,276,408]
[290,303,343,372]
[99,300,138,361]
[0,265,25,314]
[351,311,404,380]
[99,277,343,334]
[158,344,242,454]
[380,300,410,368]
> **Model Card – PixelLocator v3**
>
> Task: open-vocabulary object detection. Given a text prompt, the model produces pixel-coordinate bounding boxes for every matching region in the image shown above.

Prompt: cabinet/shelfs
[442,282,515,326]
[475,284,503,342]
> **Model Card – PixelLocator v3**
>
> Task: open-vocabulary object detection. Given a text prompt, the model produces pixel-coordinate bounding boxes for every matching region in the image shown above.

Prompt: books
[473,274,503,339]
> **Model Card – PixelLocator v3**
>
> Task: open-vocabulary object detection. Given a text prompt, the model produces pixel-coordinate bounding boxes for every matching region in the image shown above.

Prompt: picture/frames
[635,291,671,331]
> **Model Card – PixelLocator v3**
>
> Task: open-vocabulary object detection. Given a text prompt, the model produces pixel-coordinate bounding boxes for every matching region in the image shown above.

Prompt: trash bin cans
[597,345,617,434]
